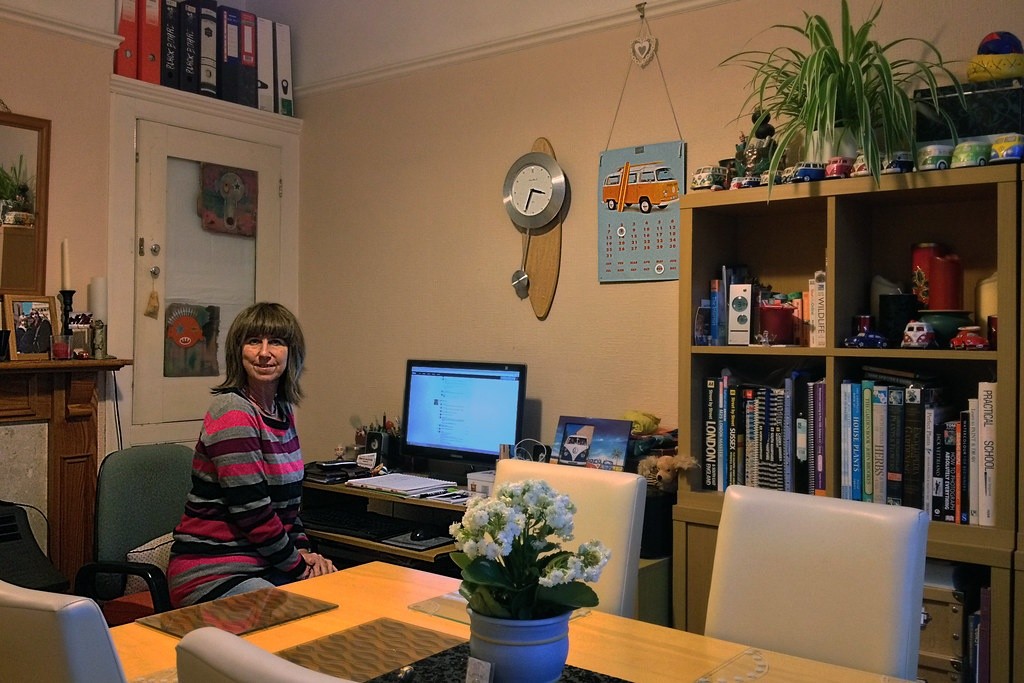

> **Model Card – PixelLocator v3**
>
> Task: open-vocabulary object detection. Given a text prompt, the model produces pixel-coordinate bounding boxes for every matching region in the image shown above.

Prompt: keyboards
[296,504,414,543]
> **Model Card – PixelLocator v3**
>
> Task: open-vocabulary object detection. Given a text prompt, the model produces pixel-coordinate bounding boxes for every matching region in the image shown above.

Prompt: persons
[30,308,52,352]
[167,303,338,606]
[15,314,36,353]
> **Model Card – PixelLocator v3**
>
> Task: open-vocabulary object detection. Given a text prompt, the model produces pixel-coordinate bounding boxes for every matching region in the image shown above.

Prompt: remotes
[315,459,357,471]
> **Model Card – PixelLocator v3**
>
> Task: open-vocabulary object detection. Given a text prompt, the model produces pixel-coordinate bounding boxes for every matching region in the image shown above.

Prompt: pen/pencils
[369,411,400,435]
[413,490,447,498]
[355,425,367,436]
[69,313,93,324]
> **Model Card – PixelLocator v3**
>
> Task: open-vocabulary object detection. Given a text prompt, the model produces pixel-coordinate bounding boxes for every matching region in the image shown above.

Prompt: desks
[108,560,926,683]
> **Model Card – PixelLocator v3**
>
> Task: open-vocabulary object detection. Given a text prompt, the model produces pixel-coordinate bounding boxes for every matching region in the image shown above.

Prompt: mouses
[410,524,442,541]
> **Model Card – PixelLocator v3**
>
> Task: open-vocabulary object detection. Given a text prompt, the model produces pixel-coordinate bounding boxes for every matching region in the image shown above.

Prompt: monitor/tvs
[398,359,528,485]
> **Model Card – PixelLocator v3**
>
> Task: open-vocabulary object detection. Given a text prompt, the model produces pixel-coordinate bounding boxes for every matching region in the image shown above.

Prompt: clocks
[503,137,568,323]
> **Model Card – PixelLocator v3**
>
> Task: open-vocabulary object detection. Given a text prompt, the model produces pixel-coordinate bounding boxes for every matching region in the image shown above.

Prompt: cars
[780,166,793,184]
[949,331,990,351]
[844,330,889,349]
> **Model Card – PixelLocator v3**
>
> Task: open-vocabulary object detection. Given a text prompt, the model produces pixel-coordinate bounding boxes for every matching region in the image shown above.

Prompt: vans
[900,320,936,349]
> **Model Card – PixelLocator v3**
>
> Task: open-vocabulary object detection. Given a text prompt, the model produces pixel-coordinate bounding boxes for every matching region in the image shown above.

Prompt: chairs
[704,484,931,680]
[0,578,131,683]
[493,458,648,615]
[75,444,195,627]
[176,627,362,683]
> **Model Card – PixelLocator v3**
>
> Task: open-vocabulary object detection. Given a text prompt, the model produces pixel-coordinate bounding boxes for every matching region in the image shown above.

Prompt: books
[710,277,726,346]
[693,366,998,529]
[304,462,371,486]
[792,272,827,347]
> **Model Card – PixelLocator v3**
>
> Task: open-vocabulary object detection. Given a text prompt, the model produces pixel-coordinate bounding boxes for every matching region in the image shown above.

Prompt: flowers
[447,479,613,619]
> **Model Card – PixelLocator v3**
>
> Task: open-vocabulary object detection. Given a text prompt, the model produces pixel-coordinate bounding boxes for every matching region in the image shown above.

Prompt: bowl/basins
[755,306,797,341]
[919,307,974,338]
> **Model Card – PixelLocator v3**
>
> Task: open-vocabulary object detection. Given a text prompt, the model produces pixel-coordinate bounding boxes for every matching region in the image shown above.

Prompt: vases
[467,602,575,683]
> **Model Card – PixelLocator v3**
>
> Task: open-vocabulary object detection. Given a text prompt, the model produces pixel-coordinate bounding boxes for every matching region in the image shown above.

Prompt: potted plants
[0,153,41,224]
[708,0,994,205]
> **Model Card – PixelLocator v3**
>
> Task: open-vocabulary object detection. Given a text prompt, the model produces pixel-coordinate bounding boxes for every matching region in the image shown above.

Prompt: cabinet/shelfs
[672,164,1024,683]
[0,351,133,589]
[303,471,467,563]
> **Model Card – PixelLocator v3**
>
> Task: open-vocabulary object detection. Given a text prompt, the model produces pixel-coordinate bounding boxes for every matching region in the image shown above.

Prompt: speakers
[533,445,551,463]
[364,431,390,470]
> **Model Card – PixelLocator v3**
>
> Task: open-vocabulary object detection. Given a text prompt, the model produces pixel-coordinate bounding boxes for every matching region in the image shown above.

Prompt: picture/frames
[4,295,60,360]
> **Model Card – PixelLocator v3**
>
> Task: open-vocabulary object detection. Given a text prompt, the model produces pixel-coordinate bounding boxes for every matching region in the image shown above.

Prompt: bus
[760,170,783,186]
[879,150,914,175]
[950,141,991,169]
[729,177,745,190]
[790,161,825,182]
[849,154,883,177]
[912,145,954,173]
[989,134,1024,165]
[690,165,728,191]
[557,434,590,466]
[823,156,856,179]
[741,177,761,188]
